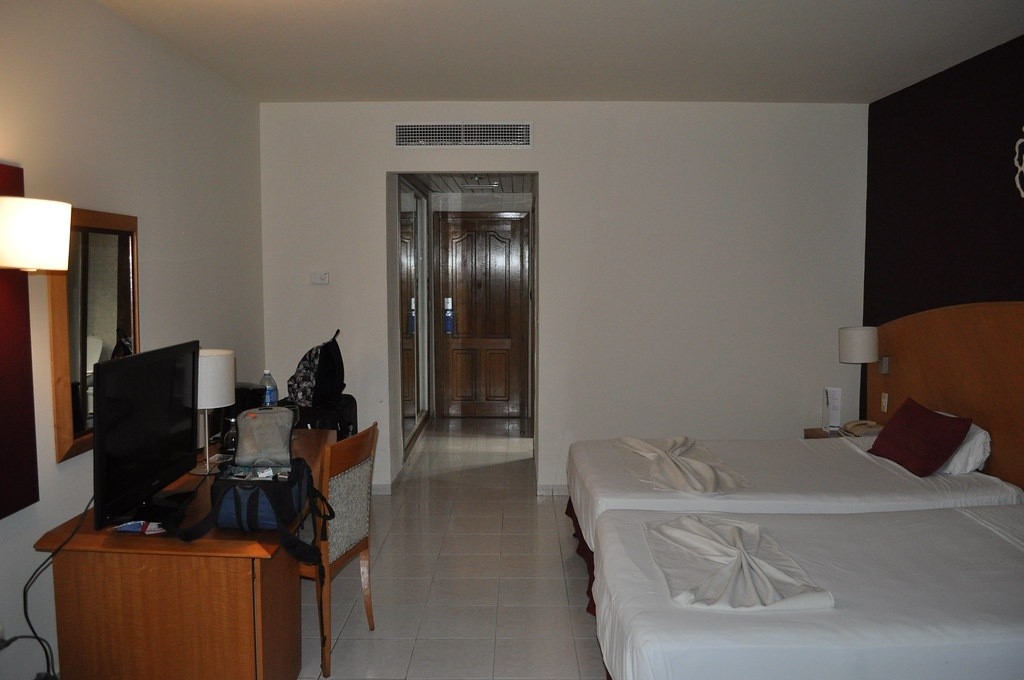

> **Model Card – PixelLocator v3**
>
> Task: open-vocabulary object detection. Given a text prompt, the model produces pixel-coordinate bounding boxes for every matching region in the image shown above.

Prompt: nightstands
[803,427,854,439]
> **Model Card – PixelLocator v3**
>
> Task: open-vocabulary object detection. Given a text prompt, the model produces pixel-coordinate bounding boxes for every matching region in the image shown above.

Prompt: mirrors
[47,207,140,464]
[395,173,433,463]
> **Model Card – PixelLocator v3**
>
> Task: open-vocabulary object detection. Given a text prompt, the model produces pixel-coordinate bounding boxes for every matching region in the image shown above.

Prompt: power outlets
[881,392,888,412]
[309,271,329,284]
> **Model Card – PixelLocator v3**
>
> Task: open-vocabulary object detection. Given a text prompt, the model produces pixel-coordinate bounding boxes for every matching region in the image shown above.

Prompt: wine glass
[224,416,239,453]
[284,405,300,440]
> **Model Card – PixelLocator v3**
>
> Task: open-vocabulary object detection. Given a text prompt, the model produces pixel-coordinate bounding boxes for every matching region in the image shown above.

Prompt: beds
[586,503,1024,680]
[563,301,1024,600]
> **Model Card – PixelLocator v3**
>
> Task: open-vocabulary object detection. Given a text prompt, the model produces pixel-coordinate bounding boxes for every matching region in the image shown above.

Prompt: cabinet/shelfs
[33,427,337,680]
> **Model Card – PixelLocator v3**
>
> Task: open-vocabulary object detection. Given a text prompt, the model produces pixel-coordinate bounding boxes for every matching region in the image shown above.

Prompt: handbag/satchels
[234,406,294,467]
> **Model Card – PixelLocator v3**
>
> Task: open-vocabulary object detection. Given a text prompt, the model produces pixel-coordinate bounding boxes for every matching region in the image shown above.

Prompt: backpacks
[209,456,334,562]
[288,329,346,407]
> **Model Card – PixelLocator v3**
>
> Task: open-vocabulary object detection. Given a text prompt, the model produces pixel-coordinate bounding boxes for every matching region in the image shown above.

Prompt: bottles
[259,370,278,406]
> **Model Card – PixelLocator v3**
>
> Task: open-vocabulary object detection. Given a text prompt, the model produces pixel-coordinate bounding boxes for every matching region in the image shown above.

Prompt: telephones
[843,420,884,437]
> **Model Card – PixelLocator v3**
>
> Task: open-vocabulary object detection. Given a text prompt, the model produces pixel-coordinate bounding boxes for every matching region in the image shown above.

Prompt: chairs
[297,423,379,678]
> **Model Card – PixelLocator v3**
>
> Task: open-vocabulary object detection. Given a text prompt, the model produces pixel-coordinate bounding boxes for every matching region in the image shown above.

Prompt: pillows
[933,411,991,475]
[866,397,973,478]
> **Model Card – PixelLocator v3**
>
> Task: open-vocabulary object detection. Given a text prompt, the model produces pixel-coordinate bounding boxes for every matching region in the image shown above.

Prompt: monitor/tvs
[89,339,199,527]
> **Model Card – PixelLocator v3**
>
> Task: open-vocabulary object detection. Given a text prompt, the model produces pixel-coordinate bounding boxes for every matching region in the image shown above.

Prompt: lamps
[187,349,235,475]
[0,195,73,271]
[839,326,889,376]
[87,334,103,373]
